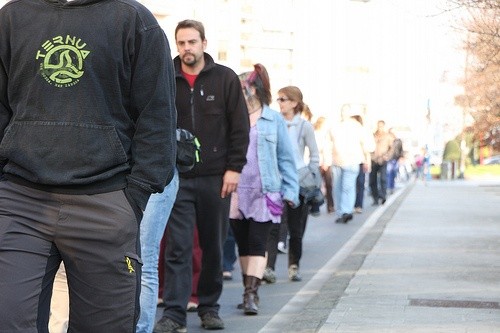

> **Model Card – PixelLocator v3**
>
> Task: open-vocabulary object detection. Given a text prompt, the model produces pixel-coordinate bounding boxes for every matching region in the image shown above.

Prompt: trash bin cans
[440,162,452,183]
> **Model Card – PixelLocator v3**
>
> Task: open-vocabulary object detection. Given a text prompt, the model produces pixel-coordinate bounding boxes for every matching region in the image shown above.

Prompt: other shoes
[288,265,300,281]
[188,302,198,312]
[335,218,342,223]
[156,297,166,307]
[341,213,352,224]
[356,208,363,213]
[262,268,276,282]
[223,271,232,280]
[278,241,287,254]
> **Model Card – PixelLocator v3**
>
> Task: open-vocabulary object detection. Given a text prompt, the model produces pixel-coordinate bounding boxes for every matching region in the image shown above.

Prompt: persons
[157,219,203,311]
[0,0,177,333]
[152,19,251,333]
[223,63,422,315]
[136,168,179,333]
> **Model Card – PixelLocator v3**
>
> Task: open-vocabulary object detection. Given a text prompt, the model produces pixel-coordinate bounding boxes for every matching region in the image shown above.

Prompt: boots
[244,275,260,314]
[237,274,248,309]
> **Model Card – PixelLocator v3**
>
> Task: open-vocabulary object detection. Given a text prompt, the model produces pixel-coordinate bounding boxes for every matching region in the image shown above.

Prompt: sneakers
[153,316,187,333]
[201,311,223,330]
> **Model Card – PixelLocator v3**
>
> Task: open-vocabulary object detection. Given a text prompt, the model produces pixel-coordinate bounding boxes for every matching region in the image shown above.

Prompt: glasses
[277,98,290,102]
